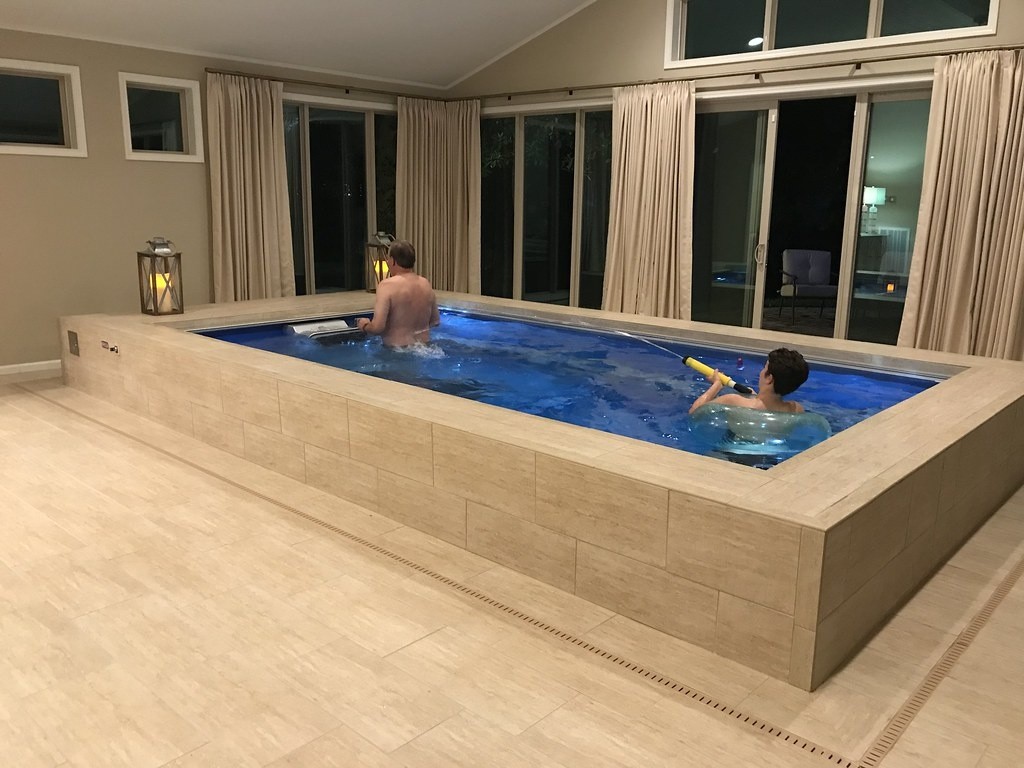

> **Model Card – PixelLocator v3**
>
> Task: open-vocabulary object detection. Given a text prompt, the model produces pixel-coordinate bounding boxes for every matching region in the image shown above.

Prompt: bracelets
[362,323,368,333]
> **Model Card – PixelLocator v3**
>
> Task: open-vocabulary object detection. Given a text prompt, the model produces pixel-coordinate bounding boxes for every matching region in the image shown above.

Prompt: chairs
[779,250,838,326]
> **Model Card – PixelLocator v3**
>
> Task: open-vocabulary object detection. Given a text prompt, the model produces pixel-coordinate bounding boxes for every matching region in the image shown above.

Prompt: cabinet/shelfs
[852,270,909,302]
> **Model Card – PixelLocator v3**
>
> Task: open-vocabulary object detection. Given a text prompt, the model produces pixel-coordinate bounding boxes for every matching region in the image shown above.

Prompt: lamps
[860,186,887,234]
[136,236,184,316]
[367,231,396,293]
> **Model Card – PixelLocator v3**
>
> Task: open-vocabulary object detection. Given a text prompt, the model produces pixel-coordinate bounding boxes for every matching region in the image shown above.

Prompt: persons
[689,347,810,415]
[359,240,440,345]
